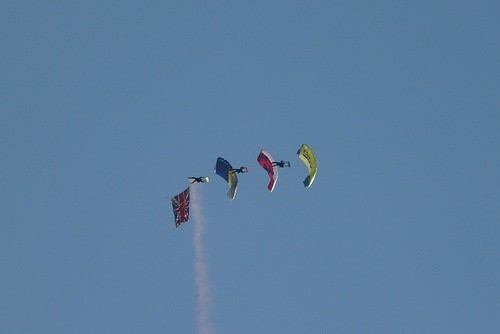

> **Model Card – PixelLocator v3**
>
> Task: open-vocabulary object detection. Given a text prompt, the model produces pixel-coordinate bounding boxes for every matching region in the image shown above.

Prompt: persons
[233,167,246,173]
[272,160,285,168]
[187,176,206,184]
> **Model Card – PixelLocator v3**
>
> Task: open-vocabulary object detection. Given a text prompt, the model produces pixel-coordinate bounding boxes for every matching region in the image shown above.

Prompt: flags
[171,187,190,228]
